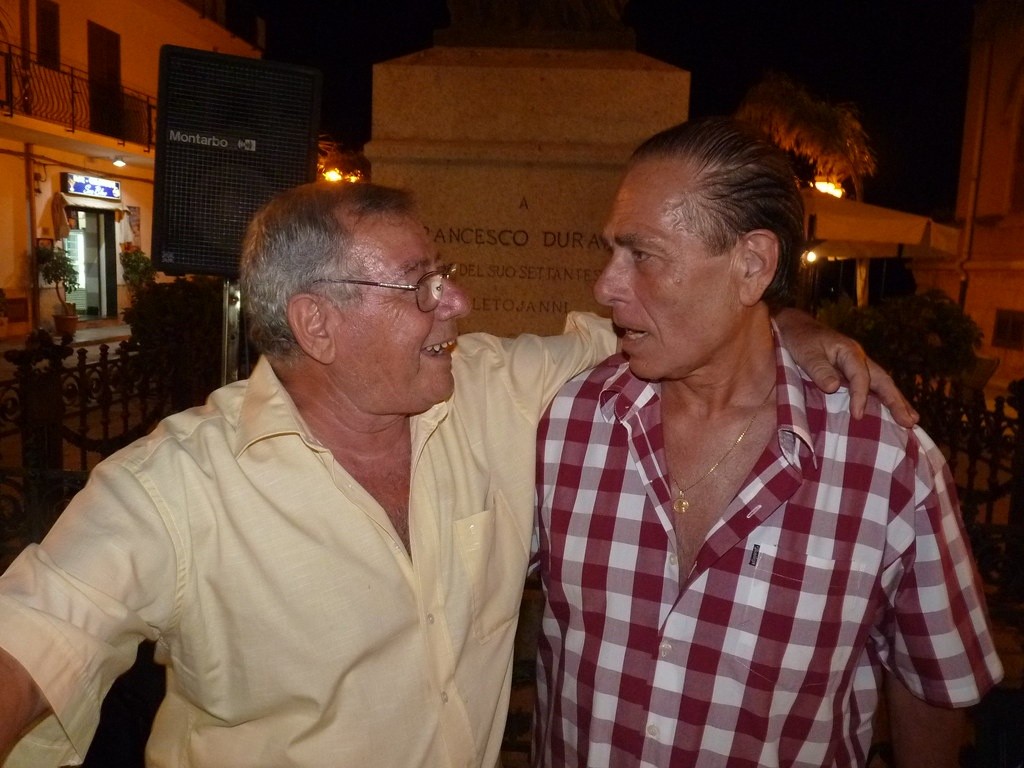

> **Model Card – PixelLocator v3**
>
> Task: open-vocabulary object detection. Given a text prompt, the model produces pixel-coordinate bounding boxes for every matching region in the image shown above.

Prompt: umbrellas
[802,186,960,259]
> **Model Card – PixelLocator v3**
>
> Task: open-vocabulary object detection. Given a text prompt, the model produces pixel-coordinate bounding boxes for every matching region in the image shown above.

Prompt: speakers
[152,44,322,277]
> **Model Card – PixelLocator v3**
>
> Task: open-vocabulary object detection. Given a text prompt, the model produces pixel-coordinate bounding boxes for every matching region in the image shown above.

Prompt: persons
[0,181,918,768]
[527,119,1006,768]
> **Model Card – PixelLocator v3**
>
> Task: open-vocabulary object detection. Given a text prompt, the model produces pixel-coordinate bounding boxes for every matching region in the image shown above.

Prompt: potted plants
[38,247,80,338]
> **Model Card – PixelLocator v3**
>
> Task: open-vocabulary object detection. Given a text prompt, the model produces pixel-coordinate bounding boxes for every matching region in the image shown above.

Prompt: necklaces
[668,380,776,514]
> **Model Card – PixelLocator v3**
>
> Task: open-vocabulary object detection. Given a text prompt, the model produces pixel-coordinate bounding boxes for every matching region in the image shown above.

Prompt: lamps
[113,157,126,167]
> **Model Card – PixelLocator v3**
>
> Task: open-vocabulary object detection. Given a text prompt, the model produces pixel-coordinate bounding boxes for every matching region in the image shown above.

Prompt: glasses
[312,261,460,314]
